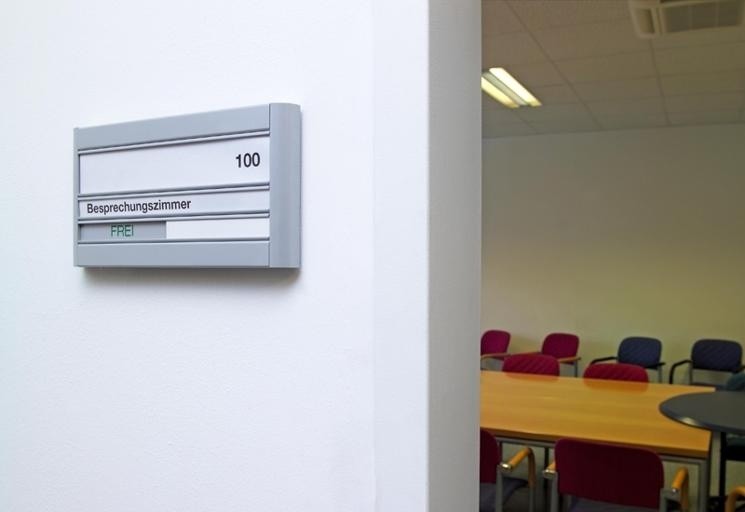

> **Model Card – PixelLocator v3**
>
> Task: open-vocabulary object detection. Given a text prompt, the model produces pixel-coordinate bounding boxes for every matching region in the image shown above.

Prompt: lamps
[480,65,543,109]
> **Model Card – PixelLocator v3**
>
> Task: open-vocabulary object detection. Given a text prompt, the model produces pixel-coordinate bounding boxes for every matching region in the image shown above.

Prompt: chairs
[500,353,563,376]
[539,332,583,378]
[478,427,540,510]
[479,330,513,370]
[718,370,745,494]
[542,435,694,512]
[669,337,744,386]
[591,336,666,382]
[583,362,651,384]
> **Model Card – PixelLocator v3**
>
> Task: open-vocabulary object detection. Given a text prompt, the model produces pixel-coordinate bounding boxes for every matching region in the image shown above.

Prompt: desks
[658,390,744,511]
[479,367,718,511]
